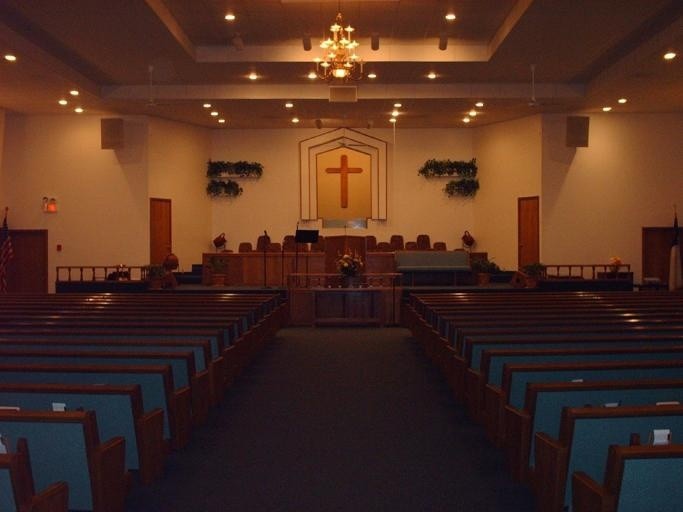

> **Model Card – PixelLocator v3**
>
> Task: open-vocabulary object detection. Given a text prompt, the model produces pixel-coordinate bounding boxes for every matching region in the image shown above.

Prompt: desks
[311,287,382,328]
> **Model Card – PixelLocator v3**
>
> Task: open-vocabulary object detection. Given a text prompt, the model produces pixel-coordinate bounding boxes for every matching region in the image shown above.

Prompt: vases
[345,274,353,288]
[608,266,620,278]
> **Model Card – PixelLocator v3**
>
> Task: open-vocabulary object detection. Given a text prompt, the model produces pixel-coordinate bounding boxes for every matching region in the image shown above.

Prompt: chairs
[222,235,464,253]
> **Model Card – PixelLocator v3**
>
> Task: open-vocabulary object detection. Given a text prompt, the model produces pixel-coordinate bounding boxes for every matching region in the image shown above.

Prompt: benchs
[394,250,471,286]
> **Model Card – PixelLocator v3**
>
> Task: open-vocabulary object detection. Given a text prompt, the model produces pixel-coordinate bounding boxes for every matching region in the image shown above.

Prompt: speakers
[101,118,123,149]
[328,85,358,103]
[567,116,590,147]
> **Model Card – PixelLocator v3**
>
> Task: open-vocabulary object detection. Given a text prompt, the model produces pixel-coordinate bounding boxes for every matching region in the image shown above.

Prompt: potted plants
[201,255,227,287]
[472,258,502,287]
[147,263,168,291]
[522,263,548,289]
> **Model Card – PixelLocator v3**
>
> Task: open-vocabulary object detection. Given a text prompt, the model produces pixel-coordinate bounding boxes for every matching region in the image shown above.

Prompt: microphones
[672,203,678,227]
[263,230,267,241]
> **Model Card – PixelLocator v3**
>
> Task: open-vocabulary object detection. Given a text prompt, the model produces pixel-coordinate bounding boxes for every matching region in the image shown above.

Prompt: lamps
[313,0,369,81]
[38,194,58,213]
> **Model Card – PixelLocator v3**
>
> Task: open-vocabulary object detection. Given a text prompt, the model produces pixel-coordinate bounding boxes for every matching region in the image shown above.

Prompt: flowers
[608,256,624,265]
[335,247,365,277]
